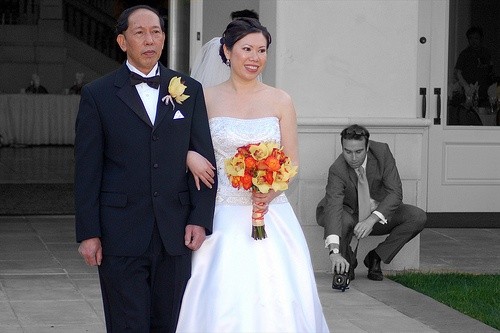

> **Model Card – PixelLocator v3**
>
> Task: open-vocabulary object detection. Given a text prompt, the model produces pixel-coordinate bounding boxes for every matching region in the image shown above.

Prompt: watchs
[329,248,340,255]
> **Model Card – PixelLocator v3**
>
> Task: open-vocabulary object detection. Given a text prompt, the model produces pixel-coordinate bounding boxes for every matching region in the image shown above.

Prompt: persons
[178,9,330,333]
[455,26,499,101]
[75,6,218,333]
[316,124,428,282]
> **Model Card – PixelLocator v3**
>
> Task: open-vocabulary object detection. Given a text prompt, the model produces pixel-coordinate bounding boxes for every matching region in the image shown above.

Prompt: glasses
[341,127,369,139]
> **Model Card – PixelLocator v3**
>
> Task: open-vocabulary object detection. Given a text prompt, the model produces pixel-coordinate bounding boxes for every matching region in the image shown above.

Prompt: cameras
[332,266,350,292]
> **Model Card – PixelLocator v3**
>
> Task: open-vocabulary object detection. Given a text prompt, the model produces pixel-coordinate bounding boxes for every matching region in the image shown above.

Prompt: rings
[361,229,365,232]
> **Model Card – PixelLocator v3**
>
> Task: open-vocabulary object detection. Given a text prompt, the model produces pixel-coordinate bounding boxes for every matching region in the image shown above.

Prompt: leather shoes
[363,249,383,281]
[348,252,358,280]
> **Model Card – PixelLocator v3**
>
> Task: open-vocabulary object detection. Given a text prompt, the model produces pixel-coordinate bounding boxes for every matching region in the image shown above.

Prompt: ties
[357,165,371,223]
[129,71,161,90]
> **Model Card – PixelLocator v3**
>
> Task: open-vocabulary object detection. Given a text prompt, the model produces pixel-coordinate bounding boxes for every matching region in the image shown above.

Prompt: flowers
[162,76,191,111]
[225,140,298,240]
